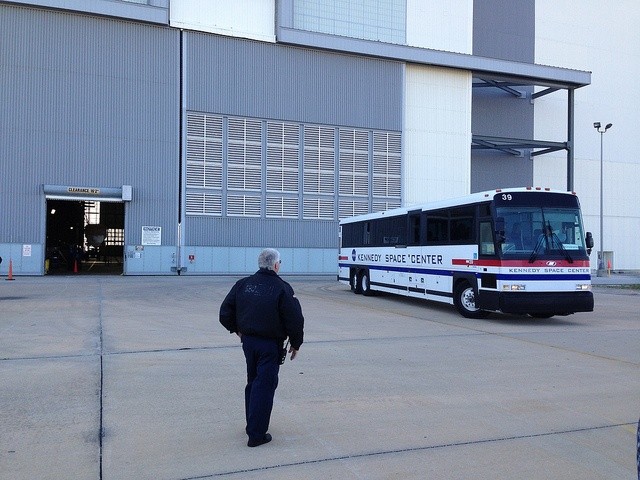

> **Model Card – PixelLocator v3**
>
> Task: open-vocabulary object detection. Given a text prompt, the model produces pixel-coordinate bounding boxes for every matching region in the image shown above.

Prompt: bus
[338,185,595,316]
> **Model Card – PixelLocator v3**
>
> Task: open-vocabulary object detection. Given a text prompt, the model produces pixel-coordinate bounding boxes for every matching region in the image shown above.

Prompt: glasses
[275,260,282,268]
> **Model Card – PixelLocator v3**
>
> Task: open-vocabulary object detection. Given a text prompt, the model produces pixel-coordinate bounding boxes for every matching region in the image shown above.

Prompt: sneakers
[247,433,273,448]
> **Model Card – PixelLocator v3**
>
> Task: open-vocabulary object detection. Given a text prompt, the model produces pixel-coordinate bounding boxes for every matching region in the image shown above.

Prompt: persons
[220,247,305,448]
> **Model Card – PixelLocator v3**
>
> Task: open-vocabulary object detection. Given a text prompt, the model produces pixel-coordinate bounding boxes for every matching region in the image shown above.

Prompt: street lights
[594,121,613,277]
